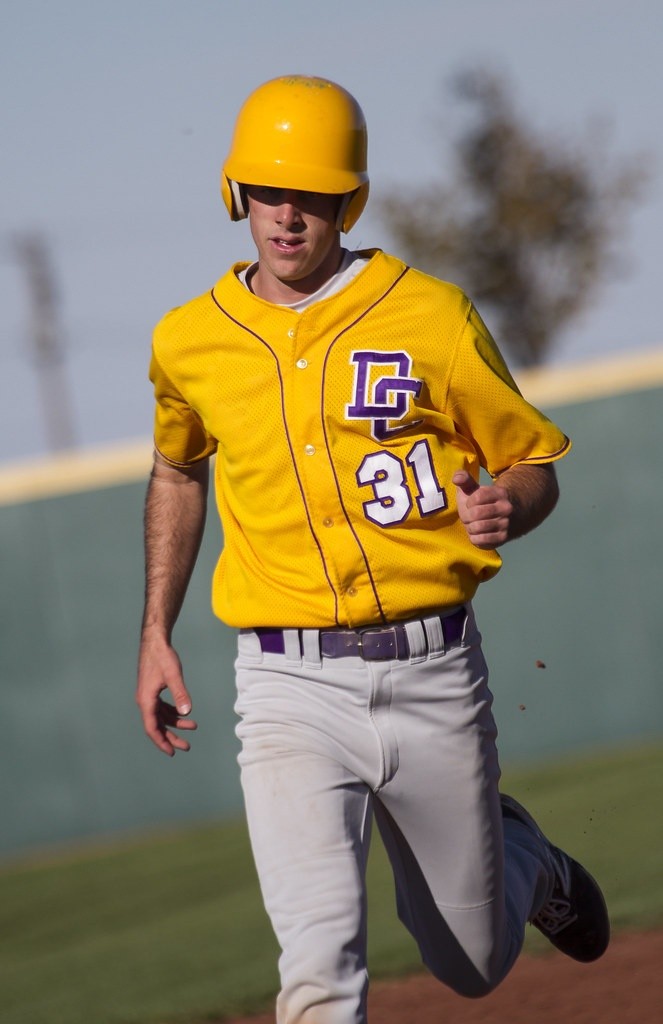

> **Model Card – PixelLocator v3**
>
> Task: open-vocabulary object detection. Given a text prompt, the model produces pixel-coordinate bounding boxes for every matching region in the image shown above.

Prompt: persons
[136,74,611,1024]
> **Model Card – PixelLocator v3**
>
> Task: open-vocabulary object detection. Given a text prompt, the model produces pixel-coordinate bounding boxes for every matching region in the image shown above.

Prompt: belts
[253,607,466,662]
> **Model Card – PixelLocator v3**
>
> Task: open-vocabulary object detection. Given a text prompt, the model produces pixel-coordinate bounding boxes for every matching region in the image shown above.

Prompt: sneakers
[500,793,610,964]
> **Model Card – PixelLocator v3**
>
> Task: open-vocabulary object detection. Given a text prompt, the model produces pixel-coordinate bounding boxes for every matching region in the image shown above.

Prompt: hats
[220,74,370,234]
